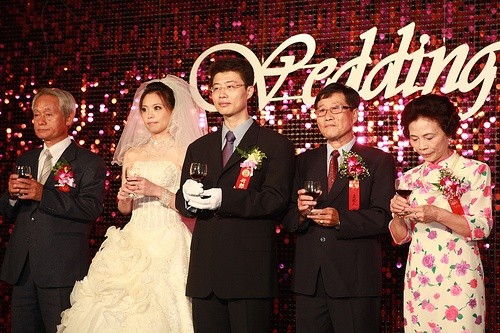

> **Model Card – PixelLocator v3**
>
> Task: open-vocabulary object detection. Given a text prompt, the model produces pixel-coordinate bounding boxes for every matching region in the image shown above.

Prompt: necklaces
[421,155,459,195]
[150,137,173,156]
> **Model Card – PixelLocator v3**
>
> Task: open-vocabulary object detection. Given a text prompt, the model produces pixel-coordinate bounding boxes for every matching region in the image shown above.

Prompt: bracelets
[9,195,17,200]
[160,192,174,206]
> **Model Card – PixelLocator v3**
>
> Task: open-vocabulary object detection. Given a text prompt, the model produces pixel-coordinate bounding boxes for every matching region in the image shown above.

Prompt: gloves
[183,179,204,201]
[188,188,222,211]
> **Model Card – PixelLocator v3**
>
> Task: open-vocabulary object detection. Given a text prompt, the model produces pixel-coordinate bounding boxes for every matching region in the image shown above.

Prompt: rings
[320,219,323,223]
[415,213,417,218]
[137,185,139,189]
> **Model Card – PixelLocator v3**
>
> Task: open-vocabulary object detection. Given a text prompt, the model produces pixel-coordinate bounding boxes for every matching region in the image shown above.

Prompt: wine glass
[304,180,322,216]
[125,167,139,199]
[190,162,208,197]
[16,166,32,197]
[395,177,413,216]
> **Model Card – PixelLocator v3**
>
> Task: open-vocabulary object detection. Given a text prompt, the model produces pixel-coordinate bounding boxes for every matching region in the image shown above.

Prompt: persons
[0,88,106,333]
[287,83,397,333]
[175,58,295,333]
[55,71,208,333]
[389,92,493,333]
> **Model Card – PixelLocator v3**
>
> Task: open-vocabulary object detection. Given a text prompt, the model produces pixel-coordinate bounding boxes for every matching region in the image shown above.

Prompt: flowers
[338,149,371,183]
[430,168,472,203]
[51,158,77,193]
[235,145,267,178]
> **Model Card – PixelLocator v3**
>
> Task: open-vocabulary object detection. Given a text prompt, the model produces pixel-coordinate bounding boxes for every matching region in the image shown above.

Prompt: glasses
[211,84,245,93]
[314,105,354,118]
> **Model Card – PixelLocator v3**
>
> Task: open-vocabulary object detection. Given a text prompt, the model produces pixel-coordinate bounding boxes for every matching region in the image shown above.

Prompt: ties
[223,131,236,168]
[328,150,341,194]
[38,149,54,186]
